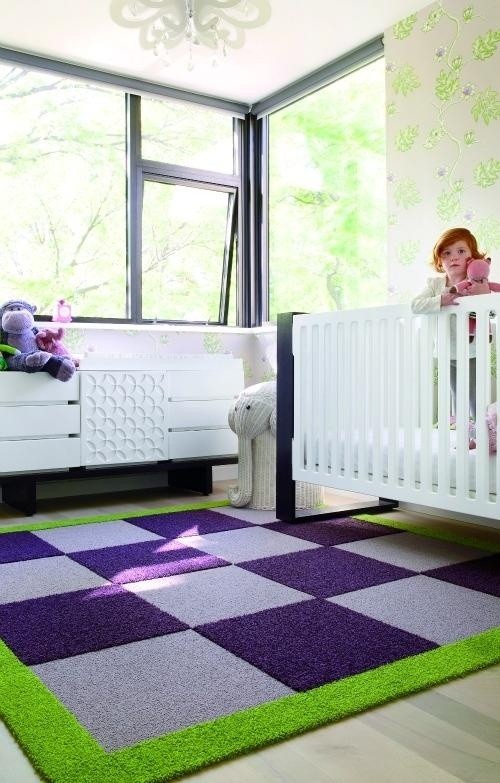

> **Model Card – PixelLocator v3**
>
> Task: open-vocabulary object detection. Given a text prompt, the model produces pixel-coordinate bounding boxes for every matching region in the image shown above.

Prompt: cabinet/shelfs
[1,357,246,516]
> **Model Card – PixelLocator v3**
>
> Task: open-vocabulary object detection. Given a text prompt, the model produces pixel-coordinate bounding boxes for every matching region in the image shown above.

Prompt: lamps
[109,0,271,71]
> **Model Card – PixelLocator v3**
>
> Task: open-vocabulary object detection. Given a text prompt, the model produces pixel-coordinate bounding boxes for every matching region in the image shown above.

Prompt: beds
[275,293,500,523]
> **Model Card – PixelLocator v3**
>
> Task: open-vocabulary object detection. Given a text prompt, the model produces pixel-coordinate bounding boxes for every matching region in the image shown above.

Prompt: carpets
[0,499,500,782]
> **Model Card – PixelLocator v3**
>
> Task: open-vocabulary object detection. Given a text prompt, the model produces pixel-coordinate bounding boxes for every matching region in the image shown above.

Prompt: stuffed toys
[449,401,497,451]
[449,257,500,343]
[0,300,80,381]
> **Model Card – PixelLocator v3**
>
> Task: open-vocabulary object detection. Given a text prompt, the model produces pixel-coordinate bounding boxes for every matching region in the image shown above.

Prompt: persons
[410,227,496,450]
